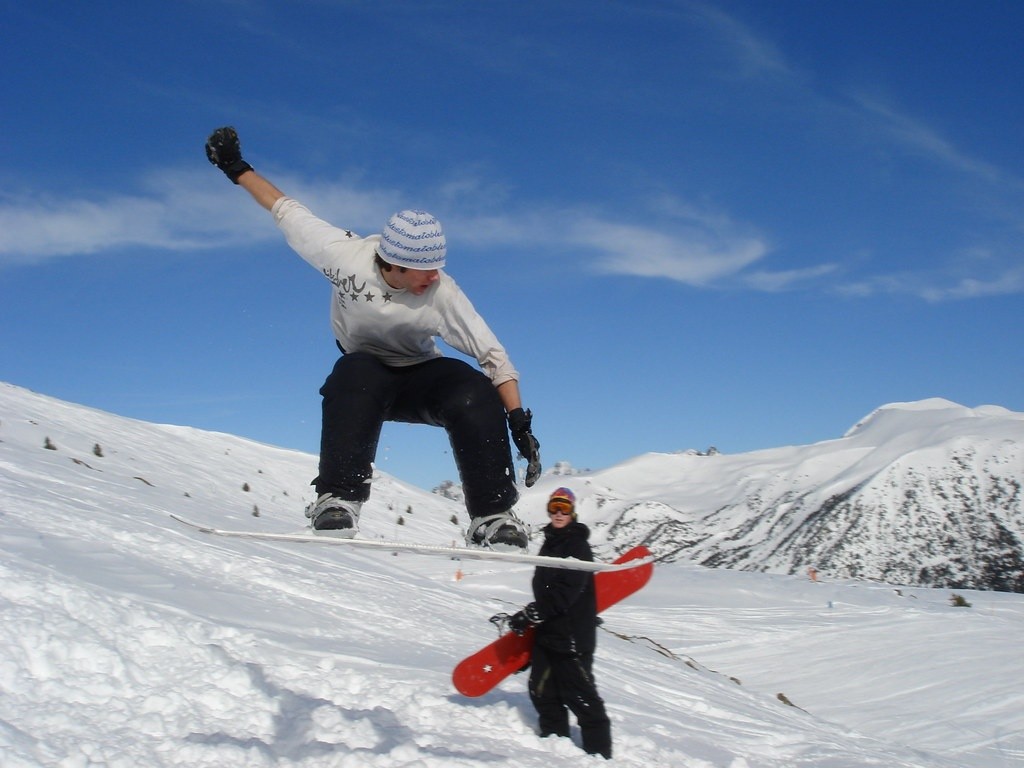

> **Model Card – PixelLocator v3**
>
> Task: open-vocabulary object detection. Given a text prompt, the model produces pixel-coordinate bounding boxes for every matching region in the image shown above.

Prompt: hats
[374,209,448,272]
[550,488,575,507]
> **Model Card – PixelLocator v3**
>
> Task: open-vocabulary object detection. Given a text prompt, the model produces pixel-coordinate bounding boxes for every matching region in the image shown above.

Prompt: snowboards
[452,546,654,698]
[169,512,655,573]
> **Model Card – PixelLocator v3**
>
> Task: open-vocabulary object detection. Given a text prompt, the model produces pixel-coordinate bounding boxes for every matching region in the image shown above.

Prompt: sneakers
[304,490,362,528]
[466,518,528,548]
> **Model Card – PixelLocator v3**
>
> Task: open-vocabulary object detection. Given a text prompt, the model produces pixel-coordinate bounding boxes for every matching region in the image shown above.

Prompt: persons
[205,127,542,548]
[508,488,612,761]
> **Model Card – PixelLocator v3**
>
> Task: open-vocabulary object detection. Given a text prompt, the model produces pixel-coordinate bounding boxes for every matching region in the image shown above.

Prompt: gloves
[507,603,545,637]
[506,408,541,488]
[204,127,255,184]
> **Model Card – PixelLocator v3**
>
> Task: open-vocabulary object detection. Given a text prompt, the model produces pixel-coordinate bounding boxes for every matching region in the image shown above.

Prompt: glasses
[547,498,574,515]
